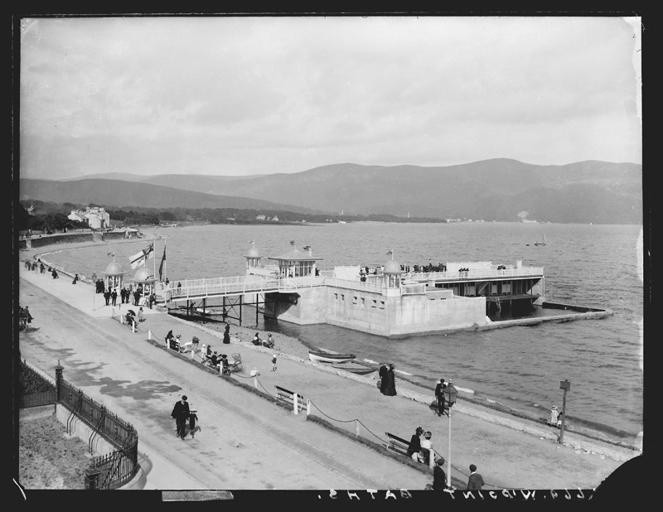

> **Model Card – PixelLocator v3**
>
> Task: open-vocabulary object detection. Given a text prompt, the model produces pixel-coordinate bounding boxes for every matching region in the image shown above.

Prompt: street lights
[440,376,458,490]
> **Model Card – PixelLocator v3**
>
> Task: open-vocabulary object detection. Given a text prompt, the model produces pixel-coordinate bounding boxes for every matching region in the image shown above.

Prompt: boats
[308,349,356,364]
[176,304,184,315]
[330,361,378,375]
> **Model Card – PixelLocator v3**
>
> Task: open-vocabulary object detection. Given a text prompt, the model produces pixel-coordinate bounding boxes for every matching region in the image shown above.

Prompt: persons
[441,380,458,417]
[548,404,568,427]
[379,363,388,393]
[17,227,509,378]
[407,426,424,462]
[188,409,202,438]
[466,463,485,490]
[383,364,397,396]
[170,395,189,440]
[435,379,446,416]
[418,431,437,464]
[432,458,448,490]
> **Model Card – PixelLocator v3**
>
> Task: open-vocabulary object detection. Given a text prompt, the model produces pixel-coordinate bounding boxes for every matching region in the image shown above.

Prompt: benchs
[382,432,429,465]
[273,384,309,413]
